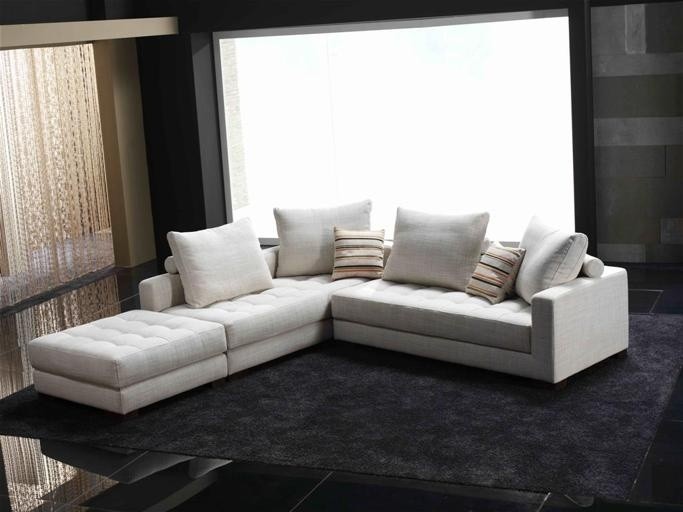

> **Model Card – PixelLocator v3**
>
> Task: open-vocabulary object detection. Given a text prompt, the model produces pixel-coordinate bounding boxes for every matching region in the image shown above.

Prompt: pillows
[381,206,490,292]
[465,241,526,305]
[273,197,372,279]
[515,209,589,304]
[330,225,386,282]
[167,215,276,309]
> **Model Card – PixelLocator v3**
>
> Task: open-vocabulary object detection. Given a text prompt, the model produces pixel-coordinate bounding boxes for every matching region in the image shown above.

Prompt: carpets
[0,305,683,506]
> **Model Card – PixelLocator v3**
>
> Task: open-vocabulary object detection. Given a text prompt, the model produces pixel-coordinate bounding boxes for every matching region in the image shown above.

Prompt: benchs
[27,309,229,417]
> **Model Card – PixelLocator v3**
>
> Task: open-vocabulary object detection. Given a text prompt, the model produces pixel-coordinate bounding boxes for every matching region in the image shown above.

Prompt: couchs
[138,239,392,377]
[331,236,630,390]
